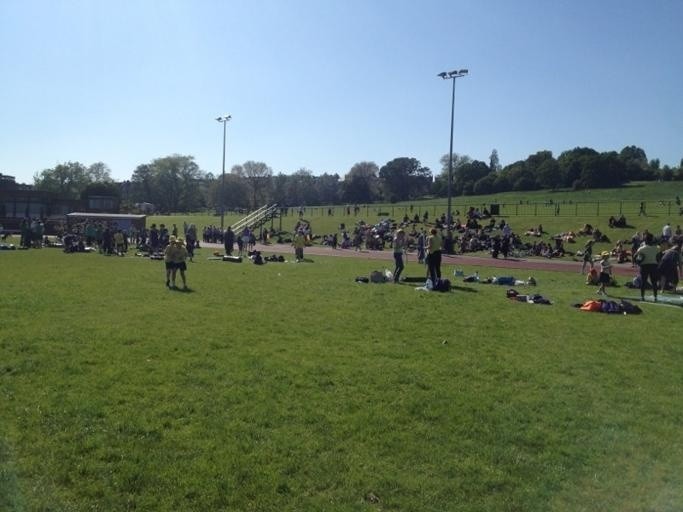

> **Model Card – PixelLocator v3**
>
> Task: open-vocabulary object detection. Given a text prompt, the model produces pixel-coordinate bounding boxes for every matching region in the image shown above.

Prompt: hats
[177,237,183,242]
[601,251,609,256]
[169,235,175,243]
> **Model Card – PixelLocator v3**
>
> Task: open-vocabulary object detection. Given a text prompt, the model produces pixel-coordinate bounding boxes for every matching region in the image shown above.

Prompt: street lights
[439,68,469,238]
[217,115,232,230]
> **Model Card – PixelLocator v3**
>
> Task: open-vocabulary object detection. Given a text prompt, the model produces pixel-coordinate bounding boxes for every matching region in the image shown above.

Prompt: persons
[19,216,138,256]
[204,204,359,265]
[135,219,198,290]
[352,203,683,302]
